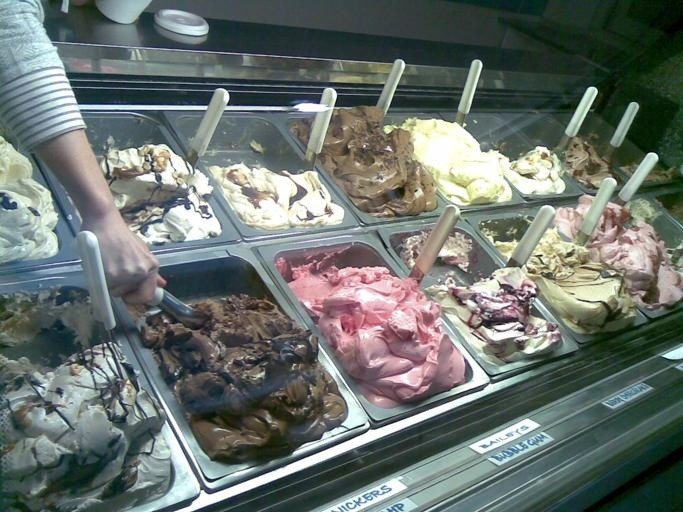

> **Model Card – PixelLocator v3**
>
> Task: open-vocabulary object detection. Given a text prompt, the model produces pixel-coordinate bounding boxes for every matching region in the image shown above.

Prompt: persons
[0,0,168,306]
